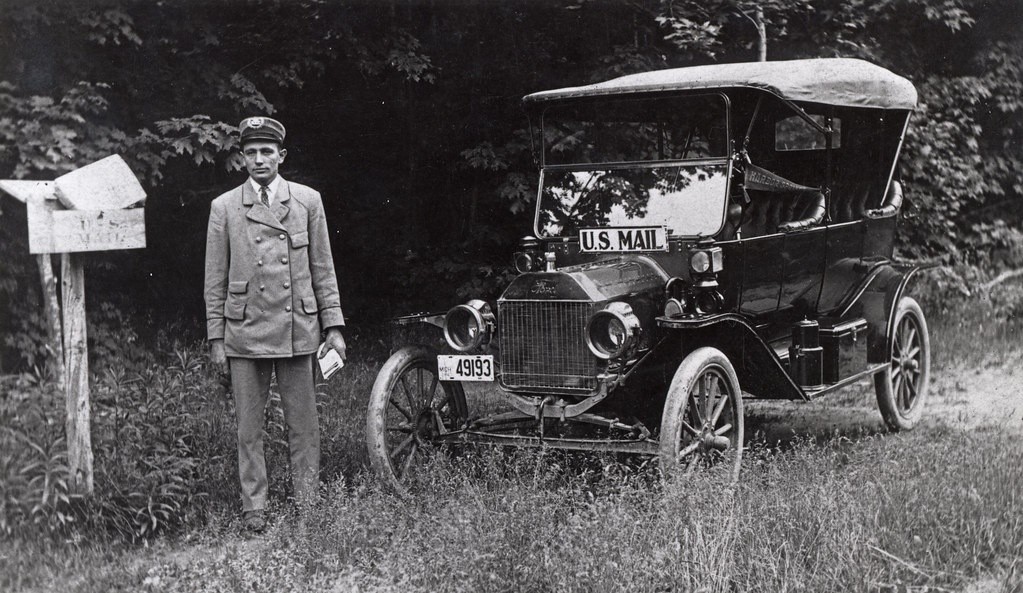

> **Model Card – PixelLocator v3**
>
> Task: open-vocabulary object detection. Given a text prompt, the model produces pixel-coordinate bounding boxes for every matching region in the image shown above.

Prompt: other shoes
[243,508,268,531]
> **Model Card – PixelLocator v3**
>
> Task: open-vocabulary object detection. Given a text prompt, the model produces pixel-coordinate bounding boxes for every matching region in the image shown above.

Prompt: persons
[204,117,346,531]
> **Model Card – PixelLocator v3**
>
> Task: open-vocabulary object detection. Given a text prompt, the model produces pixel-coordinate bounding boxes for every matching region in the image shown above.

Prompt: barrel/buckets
[788,347,823,391]
[792,321,819,348]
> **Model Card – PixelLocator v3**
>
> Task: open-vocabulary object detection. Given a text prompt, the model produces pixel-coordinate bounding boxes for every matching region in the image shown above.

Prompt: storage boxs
[0,179,147,255]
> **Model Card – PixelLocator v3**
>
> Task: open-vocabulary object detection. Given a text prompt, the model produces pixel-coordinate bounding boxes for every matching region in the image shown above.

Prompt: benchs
[743,167,903,231]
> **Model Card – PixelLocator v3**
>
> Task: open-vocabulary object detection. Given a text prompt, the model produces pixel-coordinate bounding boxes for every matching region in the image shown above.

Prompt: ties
[258,186,270,208]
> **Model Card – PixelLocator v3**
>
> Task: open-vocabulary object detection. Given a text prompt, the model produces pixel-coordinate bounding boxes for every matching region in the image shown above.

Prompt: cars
[365,57,941,503]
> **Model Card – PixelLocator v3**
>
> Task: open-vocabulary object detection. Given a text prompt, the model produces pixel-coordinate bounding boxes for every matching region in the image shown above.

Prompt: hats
[238,116,286,146]
